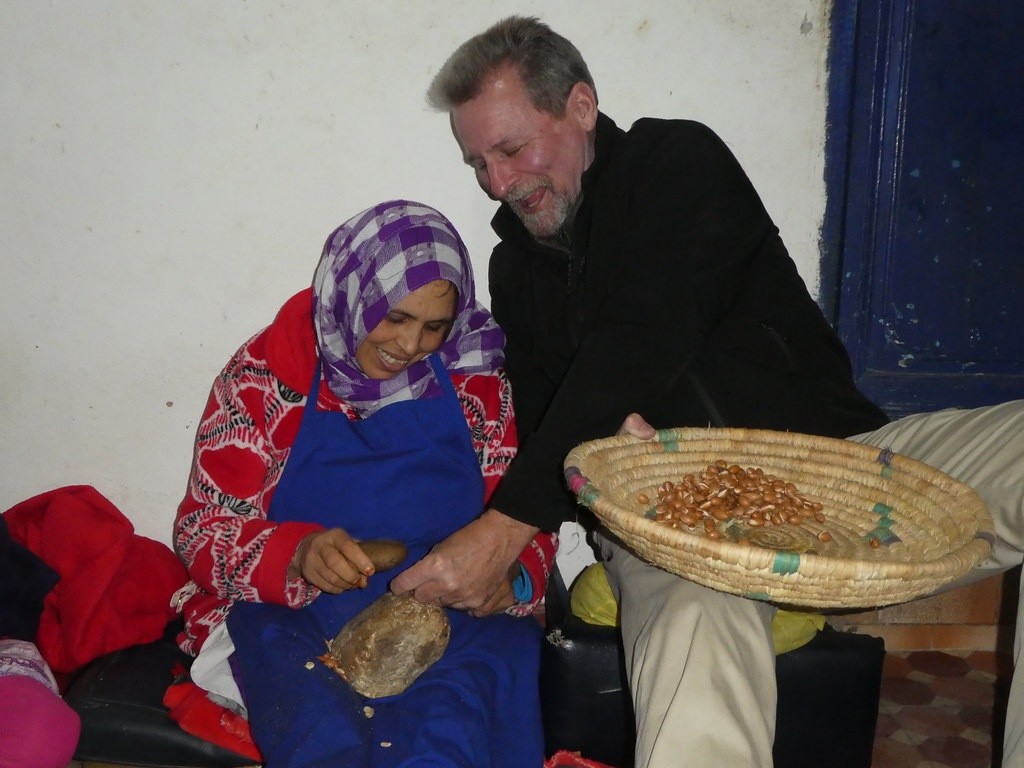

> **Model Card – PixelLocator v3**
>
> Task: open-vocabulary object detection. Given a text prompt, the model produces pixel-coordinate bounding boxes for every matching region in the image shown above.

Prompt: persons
[389,15,1024,768]
[173,198,559,768]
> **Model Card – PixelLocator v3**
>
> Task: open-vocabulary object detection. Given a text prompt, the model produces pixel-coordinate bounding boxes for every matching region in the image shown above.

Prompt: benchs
[56,582,880,768]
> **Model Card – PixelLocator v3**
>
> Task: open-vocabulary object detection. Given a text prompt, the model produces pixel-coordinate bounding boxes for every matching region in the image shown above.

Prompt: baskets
[562,427,997,609]
